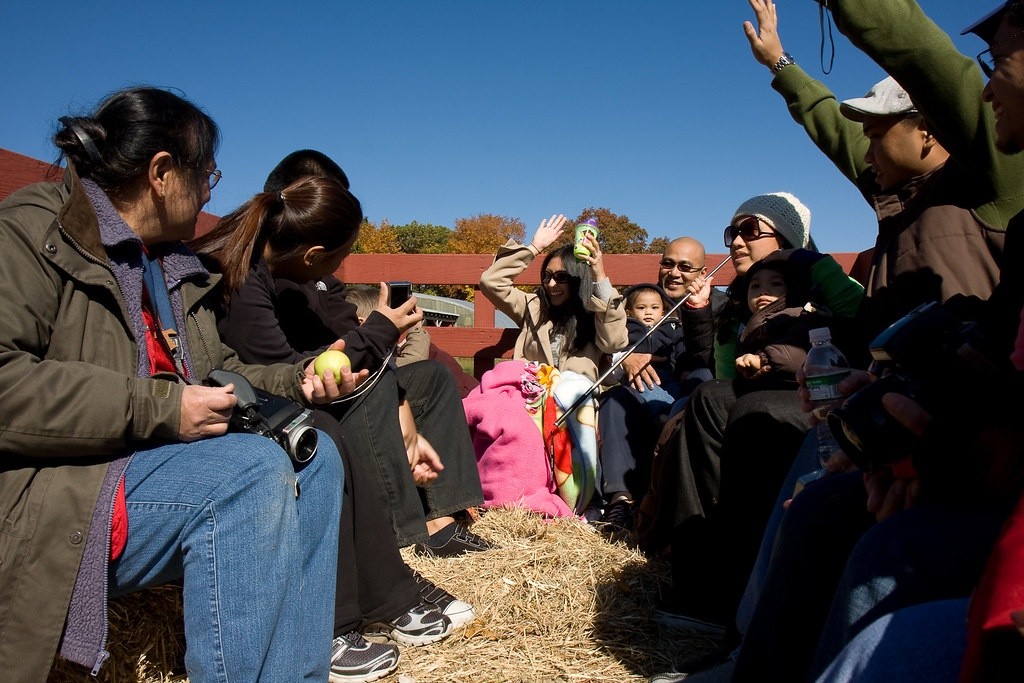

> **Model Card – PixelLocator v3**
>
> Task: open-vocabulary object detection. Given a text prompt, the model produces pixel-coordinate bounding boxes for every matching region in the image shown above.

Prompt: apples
[313,349,351,386]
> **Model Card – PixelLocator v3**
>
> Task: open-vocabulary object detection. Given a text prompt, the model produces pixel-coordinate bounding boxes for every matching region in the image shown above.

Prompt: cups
[573,225,599,258]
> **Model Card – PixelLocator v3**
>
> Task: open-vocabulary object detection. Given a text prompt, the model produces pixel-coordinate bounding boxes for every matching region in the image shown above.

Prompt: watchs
[770,51,796,74]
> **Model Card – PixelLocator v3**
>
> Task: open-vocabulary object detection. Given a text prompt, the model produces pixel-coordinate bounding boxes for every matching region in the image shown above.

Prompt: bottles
[804,328,855,472]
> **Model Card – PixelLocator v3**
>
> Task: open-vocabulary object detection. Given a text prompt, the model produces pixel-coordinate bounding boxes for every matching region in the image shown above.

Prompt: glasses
[541,270,573,284]
[659,259,702,274]
[172,156,223,190]
[723,215,776,249]
[976,34,1022,77]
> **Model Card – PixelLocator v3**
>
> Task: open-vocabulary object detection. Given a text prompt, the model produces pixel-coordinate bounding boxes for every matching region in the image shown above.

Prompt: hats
[961,0,1024,45]
[841,73,917,123]
[729,191,811,250]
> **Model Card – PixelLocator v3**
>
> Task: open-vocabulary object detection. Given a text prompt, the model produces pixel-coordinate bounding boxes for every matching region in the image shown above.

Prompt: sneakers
[628,557,673,600]
[327,631,400,683]
[598,499,637,541]
[414,523,502,558]
[404,565,474,628]
[363,602,452,647]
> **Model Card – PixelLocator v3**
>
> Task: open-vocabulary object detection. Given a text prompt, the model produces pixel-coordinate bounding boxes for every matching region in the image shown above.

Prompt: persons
[183,171,453,683]
[263,148,503,629]
[0,87,346,683]
[646,0,1024,683]
[480,213,628,516]
[599,237,727,537]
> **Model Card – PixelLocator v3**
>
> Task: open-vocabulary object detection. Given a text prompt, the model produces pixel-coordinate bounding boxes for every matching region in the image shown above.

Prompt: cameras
[826,293,1024,474]
[201,378,318,462]
[386,281,412,309]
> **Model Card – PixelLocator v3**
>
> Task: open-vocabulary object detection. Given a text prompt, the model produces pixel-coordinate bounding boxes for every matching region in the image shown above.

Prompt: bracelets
[531,243,540,253]
